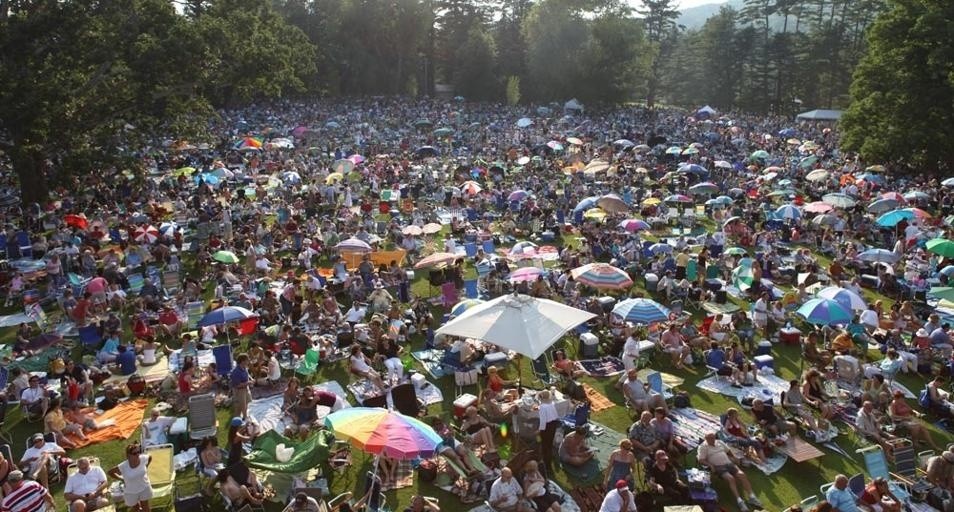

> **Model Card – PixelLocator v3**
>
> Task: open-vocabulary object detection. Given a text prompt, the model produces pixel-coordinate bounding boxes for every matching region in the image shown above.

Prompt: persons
[1,93,953,510]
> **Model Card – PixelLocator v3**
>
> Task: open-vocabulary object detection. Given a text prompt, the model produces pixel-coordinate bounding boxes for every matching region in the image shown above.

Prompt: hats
[231,418,245,427]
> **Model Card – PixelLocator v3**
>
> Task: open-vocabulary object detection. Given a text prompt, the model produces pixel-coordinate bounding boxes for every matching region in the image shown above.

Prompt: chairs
[1,212,950,512]
